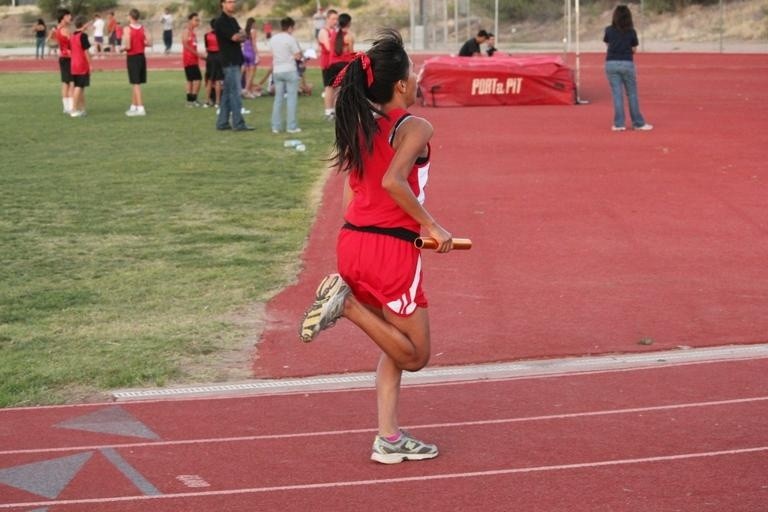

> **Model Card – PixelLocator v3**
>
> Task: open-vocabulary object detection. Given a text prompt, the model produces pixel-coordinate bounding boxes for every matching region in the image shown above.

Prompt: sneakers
[124,109,146,117]
[63,107,86,118]
[272,128,280,134]
[611,122,626,131]
[186,101,221,108]
[326,109,336,119]
[297,272,353,343]
[286,128,302,134]
[634,122,654,130]
[371,428,439,465]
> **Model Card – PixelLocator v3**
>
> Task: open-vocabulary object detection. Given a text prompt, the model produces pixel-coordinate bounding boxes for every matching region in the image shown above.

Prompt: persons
[603,5,653,130]
[298,30,452,463]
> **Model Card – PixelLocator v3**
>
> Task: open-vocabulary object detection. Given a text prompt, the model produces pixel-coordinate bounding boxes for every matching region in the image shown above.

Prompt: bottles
[296,144,305,151]
[284,140,303,148]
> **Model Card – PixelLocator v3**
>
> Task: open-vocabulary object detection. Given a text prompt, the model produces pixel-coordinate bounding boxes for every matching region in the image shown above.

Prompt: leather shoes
[216,123,232,130]
[233,125,257,132]
